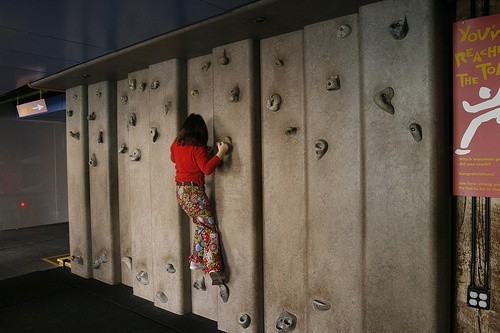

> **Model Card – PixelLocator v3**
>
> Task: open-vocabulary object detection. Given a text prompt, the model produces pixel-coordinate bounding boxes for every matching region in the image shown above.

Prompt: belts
[174,181,202,187]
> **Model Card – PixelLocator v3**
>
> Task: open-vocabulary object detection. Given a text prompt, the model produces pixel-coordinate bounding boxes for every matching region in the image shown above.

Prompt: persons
[170,112,231,285]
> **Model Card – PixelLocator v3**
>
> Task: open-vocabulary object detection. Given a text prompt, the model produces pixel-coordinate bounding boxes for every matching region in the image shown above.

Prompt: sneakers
[209,268,224,287]
[189,260,204,270]
